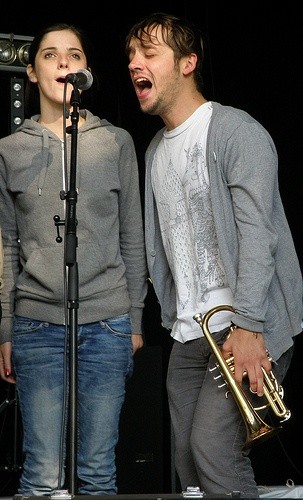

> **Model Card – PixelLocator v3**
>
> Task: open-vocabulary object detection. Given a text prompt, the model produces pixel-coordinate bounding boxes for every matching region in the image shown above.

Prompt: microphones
[66,68,93,90]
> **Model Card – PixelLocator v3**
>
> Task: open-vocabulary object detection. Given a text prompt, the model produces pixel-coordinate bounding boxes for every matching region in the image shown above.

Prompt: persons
[0,22,149,495]
[127,13,303,500]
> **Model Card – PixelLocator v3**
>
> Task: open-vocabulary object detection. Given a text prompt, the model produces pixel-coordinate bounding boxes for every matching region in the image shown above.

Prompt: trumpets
[193,305,291,447]
